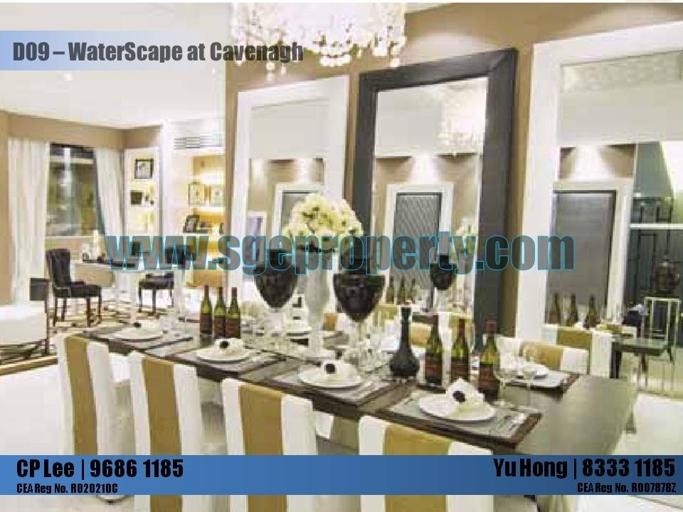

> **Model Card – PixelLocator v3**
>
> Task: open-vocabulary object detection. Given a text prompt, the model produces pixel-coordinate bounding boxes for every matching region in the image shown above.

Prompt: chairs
[0,248,683,512]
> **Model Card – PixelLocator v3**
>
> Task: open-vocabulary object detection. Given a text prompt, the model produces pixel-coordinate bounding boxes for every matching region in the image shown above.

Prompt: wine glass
[490,345,518,412]
[332,271,384,363]
[524,342,540,407]
[251,265,298,349]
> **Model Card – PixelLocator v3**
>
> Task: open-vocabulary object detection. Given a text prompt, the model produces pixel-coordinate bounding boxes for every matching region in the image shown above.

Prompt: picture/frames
[181,215,199,233]
[133,158,154,180]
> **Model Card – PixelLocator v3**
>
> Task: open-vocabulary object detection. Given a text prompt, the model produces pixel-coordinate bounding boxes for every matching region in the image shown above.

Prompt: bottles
[386,276,418,307]
[451,318,472,386]
[564,294,577,327]
[584,296,598,328]
[476,318,502,402]
[549,291,567,327]
[423,314,444,387]
[386,308,419,378]
[197,284,242,343]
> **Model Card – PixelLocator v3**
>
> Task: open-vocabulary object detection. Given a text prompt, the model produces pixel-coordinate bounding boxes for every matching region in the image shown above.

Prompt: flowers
[278,184,365,248]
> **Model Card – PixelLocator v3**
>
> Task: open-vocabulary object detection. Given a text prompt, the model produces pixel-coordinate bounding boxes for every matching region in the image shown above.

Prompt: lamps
[229,3,408,84]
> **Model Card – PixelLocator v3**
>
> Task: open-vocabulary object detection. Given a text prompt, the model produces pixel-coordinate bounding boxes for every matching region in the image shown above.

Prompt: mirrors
[225,71,352,319]
[352,45,518,354]
[510,18,681,512]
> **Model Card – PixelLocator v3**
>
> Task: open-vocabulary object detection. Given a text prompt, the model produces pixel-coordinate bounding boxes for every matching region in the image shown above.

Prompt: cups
[323,312,338,332]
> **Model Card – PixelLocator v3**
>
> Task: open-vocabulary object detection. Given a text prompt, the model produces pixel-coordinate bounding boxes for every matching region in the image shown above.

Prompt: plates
[254,319,311,335]
[415,394,497,421]
[196,345,250,365]
[111,325,160,343]
[512,361,548,378]
[295,365,363,388]
